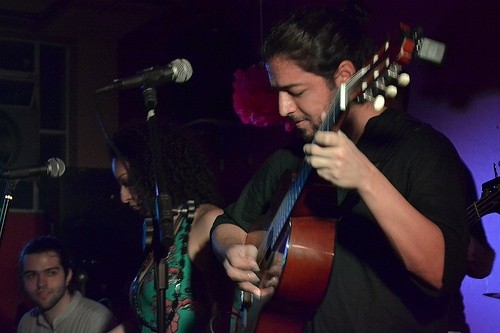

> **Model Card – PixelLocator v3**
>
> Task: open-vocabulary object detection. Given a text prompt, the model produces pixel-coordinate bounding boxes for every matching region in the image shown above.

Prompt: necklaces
[132,215,183,296]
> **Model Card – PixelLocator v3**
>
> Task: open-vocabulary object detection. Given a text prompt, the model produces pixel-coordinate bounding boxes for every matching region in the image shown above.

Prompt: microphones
[0,157,66,179]
[97,58,193,95]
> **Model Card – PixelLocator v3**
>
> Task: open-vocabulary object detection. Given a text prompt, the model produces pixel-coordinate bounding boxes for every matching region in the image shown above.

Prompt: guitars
[466,160,500,223]
[230,22,419,333]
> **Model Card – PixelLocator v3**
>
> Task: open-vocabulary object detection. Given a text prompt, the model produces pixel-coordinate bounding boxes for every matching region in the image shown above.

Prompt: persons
[210,15,495,333]
[108,127,225,333]
[17,235,110,333]
